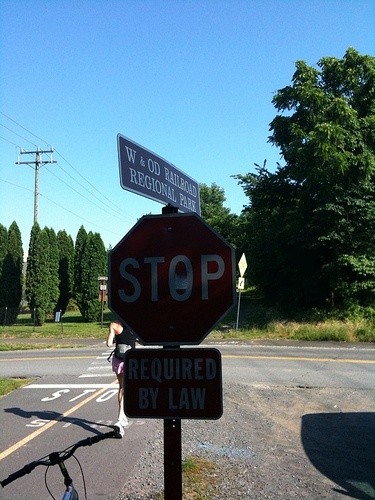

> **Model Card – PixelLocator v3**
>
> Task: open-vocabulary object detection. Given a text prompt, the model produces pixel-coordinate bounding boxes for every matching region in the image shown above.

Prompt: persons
[107,319,138,438]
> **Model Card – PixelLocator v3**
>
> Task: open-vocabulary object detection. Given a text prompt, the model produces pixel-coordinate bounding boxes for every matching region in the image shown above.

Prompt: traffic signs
[115,133,203,215]
[123,346,223,422]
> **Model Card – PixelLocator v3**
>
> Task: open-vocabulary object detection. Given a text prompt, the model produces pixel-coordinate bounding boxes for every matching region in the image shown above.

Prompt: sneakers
[113,421,125,438]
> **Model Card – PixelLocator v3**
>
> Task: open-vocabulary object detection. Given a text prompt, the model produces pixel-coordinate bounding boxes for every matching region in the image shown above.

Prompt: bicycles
[0,430,123,500]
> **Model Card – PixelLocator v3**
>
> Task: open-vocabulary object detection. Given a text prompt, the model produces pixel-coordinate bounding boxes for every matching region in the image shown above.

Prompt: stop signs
[106,211,238,346]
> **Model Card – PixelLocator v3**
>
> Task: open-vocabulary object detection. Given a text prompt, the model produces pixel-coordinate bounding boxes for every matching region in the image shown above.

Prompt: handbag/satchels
[107,343,132,362]
